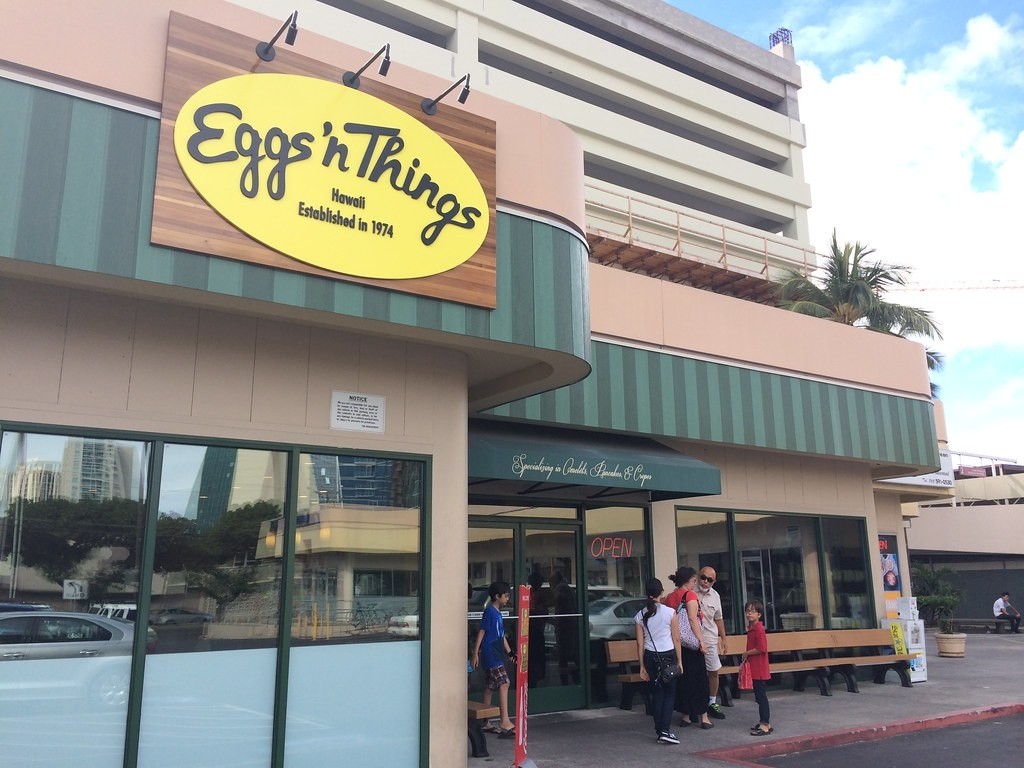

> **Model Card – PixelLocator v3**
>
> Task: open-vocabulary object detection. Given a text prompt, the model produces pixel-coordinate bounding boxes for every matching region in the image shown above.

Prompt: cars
[0,601,157,662]
[388,611,419,640]
[149,607,216,627]
[582,596,649,641]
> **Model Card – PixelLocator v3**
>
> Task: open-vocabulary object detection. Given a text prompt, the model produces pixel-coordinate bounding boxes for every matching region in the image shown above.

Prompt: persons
[694,566,731,719]
[992,592,1023,633]
[632,577,683,745]
[664,567,714,728]
[470,581,516,740]
[741,599,773,736]
[524,570,581,687]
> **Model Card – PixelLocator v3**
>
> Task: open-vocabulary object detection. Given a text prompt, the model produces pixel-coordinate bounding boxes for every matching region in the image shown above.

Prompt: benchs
[467,701,501,758]
[939,619,1010,635]
[606,628,917,709]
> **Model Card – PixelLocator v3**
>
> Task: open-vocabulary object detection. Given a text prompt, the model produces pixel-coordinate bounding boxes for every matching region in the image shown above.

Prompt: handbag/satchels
[659,665,679,684]
[674,591,703,650]
[737,657,753,690]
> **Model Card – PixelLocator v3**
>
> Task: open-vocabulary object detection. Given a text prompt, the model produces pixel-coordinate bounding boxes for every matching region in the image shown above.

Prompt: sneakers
[707,703,725,719]
[657,731,680,744]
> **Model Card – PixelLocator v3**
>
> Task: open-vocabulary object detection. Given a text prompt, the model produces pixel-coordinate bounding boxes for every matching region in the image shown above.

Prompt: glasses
[699,573,715,583]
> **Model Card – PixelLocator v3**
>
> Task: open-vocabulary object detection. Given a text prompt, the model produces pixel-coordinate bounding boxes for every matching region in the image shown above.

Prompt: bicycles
[345,601,408,637]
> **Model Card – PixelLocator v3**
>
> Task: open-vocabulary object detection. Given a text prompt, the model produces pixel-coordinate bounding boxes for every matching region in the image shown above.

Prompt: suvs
[468,584,635,661]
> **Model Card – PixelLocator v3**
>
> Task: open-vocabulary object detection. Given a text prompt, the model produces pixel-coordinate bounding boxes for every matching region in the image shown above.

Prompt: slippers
[750,729,770,736]
[482,726,501,734]
[751,723,773,732]
[499,726,517,738]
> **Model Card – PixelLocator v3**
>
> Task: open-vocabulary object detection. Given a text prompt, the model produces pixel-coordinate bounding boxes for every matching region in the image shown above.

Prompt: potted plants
[934,606,967,658]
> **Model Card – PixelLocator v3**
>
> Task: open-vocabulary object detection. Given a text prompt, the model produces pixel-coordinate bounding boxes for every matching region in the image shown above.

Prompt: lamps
[342,43,392,91]
[255,9,298,62]
[420,72,471,116]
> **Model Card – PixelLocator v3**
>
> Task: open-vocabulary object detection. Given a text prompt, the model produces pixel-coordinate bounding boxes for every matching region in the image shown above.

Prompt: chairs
[37,620,54,639]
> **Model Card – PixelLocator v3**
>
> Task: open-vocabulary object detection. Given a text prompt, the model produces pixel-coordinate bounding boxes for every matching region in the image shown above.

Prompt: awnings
[468,418,723,503]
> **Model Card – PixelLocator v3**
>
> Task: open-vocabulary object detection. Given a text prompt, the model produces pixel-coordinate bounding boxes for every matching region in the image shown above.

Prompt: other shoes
[680,720,690,727]
[702,721,714,729]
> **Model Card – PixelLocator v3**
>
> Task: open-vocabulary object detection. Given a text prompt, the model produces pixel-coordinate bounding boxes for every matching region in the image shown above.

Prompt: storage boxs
[881,618,925,648]
[881,648,927,682]
[896,597,918,620]
